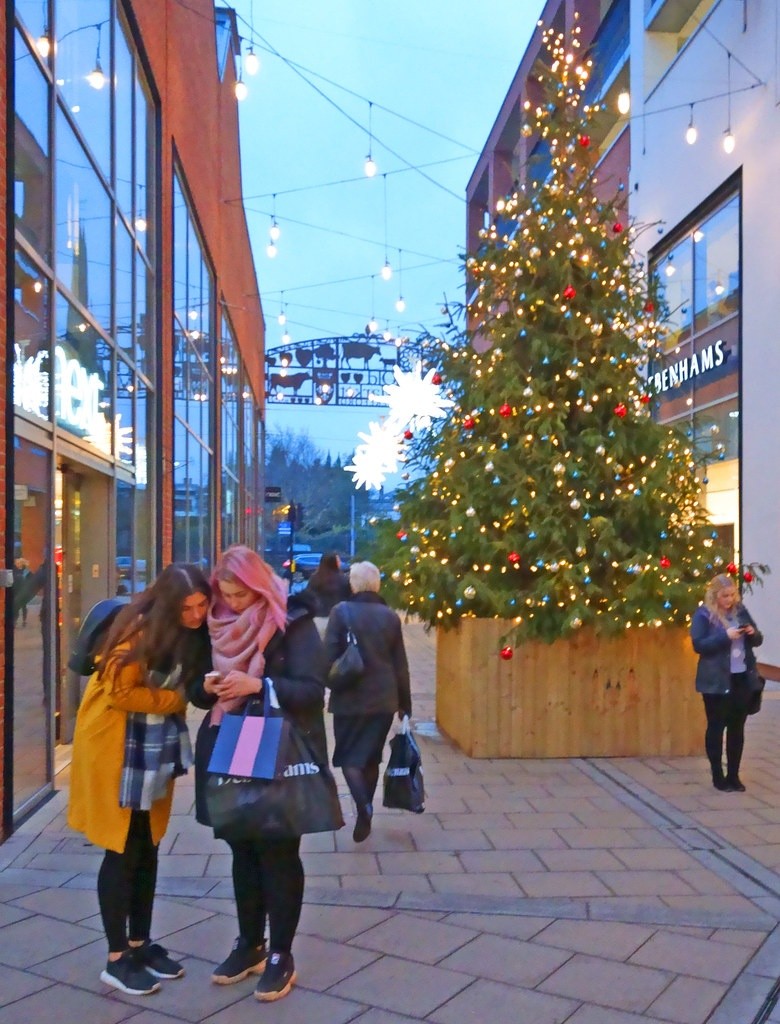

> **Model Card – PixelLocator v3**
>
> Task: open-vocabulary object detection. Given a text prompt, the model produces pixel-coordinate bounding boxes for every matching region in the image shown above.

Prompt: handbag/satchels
[383,715,427,814]
[747,677,766,714]
[324,602,366,691]
[206,679,342,842]
[204,681,290,782]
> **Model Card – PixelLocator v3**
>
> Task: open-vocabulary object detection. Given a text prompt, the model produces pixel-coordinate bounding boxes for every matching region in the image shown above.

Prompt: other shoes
[726,775,746,792]
[713,775,728,790]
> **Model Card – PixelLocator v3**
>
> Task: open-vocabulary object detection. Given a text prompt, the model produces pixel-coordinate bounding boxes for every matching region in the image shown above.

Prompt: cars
[116,556,132,578]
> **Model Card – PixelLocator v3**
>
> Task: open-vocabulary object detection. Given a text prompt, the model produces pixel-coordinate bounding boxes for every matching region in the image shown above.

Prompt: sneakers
[254,951,297,1001]
[129,940,185,979]
[211,935,268,985]
[100,955,161,994]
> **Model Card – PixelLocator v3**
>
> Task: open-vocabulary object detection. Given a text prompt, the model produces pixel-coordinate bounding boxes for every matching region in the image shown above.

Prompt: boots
[344,767,375,842]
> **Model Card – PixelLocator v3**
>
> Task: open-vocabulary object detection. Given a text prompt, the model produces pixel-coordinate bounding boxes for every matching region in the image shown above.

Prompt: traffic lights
[283,501,295,521]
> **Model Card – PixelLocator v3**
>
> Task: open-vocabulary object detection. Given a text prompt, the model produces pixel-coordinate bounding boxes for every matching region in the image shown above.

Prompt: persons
[14,558,34,627]
[67,563,211,996]
[184,547,325,1002]
[322,560,413,842]
[689,574,763,791]
[307,551,353,714]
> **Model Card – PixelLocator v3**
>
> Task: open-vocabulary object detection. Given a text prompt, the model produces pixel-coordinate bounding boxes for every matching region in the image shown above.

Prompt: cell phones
[737,624,748,633]
[205,673,223,684]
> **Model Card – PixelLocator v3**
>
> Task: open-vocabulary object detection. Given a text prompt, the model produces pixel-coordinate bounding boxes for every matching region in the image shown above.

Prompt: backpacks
[68,597,132,677]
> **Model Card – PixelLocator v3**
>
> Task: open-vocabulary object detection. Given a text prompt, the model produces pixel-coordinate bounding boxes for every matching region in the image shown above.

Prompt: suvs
[280,552,350,579]
